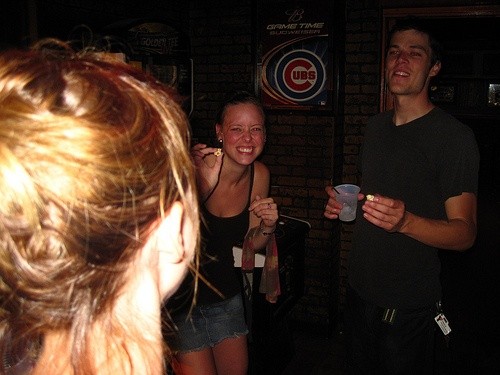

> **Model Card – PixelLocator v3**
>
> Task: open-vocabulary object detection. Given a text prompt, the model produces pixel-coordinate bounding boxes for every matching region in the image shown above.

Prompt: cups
[333,184,360,222]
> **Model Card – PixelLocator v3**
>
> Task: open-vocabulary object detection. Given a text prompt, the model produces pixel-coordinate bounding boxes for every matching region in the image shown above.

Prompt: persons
[0,39,200,374]
[159,91,280,374]
[323,16,481,375]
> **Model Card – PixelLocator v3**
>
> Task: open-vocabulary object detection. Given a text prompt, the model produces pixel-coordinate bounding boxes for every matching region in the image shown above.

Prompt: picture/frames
[379,6,500,121]
[121,17,195,117]
[251,4,342,118]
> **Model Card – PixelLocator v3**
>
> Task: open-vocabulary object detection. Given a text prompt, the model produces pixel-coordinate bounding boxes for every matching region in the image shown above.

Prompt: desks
[244,212,312,343]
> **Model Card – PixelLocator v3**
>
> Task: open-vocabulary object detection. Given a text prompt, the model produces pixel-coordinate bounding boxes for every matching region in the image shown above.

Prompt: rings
[267,201,272,210]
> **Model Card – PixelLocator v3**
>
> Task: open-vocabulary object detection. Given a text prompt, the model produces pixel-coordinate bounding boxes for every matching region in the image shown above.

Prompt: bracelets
[259,219,278,238]
